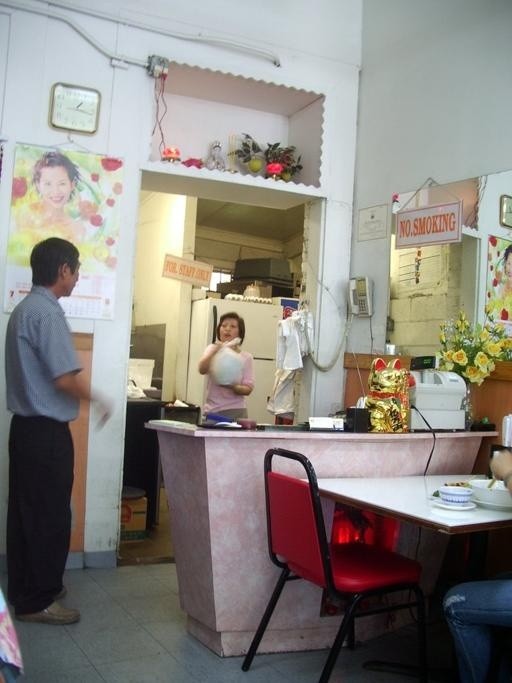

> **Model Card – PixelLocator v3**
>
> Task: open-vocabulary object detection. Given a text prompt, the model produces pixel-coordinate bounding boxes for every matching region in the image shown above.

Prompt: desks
[121,398,161,531]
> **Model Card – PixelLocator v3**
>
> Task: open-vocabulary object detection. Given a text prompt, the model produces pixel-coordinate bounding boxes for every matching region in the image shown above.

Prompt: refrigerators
[185,297,285,425]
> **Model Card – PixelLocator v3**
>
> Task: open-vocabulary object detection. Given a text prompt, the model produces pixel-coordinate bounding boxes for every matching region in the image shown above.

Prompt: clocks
[500,194,512,228]
[48,82,100,134]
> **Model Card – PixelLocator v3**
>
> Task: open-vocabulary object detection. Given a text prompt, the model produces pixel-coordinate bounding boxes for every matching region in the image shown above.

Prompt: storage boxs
[120,497,148,543]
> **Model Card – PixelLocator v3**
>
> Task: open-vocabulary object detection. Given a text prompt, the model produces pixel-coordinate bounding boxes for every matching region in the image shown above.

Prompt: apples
[249,158,263,173]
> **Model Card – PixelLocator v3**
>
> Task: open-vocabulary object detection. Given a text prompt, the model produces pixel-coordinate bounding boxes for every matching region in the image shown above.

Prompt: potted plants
[229,133,302,181]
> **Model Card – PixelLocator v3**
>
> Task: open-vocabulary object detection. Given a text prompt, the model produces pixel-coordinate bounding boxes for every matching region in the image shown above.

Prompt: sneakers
[13,583,81,625]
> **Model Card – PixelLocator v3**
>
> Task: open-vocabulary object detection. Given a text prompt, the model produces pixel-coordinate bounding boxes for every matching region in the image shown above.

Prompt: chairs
[242,449,426,683]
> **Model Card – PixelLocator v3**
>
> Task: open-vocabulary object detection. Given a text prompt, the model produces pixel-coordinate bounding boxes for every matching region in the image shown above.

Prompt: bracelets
[502,472,512,487]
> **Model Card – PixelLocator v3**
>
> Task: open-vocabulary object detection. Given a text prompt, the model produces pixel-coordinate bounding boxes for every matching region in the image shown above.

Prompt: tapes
[239,418,257,430]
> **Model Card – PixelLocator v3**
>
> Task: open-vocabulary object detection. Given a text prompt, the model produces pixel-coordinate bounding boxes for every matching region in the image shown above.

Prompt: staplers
[205,415,234,429]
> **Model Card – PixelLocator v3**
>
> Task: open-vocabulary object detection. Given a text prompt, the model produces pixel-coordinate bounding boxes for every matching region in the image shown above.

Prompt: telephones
[347,276,373,318]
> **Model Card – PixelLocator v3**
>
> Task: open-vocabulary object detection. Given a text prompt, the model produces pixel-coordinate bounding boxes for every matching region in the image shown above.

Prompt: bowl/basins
[439,486,473,506]
[469,479,512,505]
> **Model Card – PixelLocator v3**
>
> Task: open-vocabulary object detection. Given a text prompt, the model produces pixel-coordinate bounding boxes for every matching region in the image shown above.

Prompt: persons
[204,140,228,170]
[496,246,512,321]
[443,447,512,682]
[2,237,113,626]
[198,311,254,421]
[9,152,88,247]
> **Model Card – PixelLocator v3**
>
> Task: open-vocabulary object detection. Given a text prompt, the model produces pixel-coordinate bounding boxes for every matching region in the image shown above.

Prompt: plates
[432,500,476,511]
[470,498,512,512]
